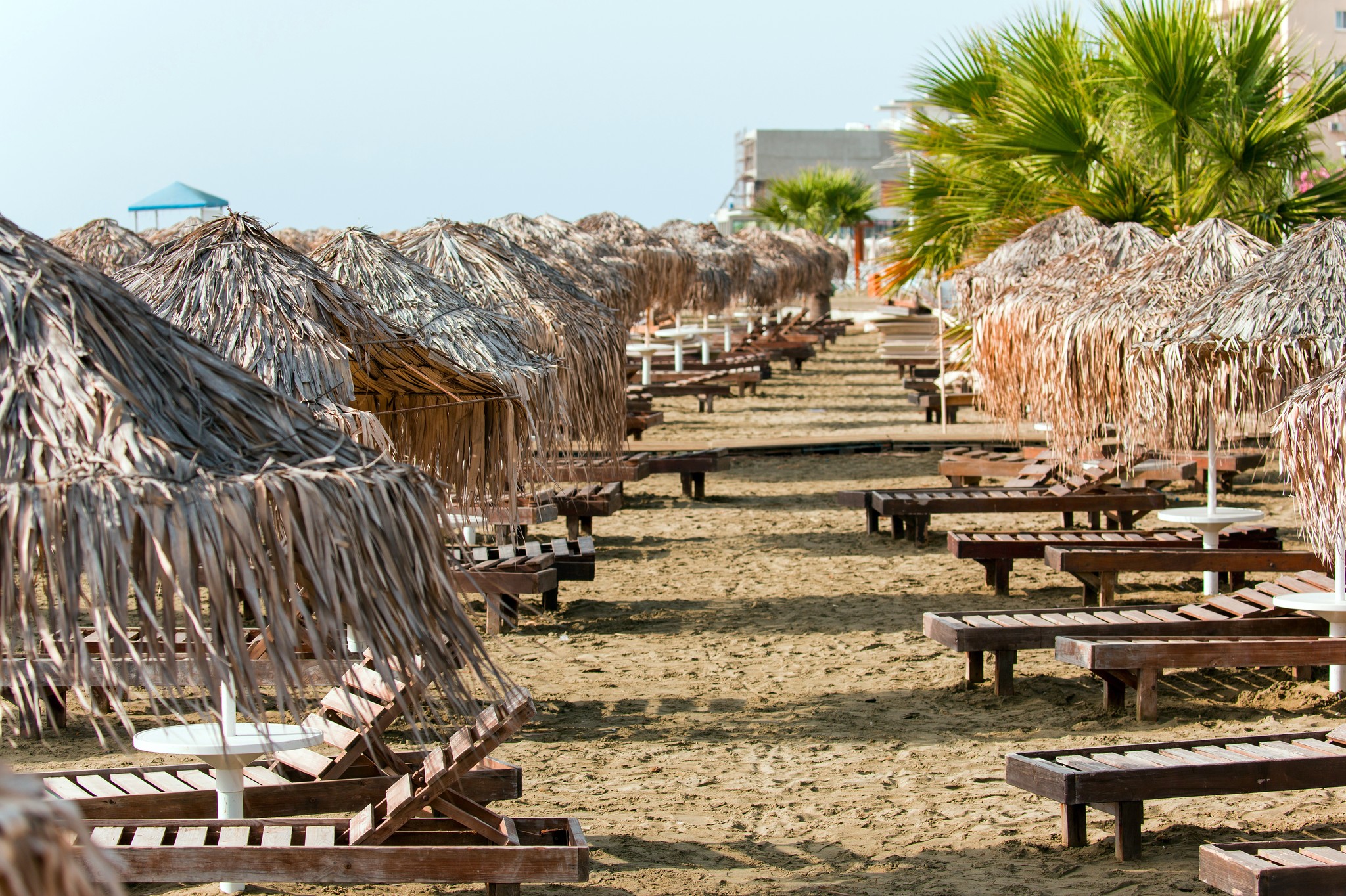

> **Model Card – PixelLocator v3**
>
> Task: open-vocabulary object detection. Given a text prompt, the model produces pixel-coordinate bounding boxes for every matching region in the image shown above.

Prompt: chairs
[0,295,1346,896]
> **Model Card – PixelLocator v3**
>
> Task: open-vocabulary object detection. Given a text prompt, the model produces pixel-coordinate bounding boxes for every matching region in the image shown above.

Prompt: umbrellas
[0,195,1346,896]
